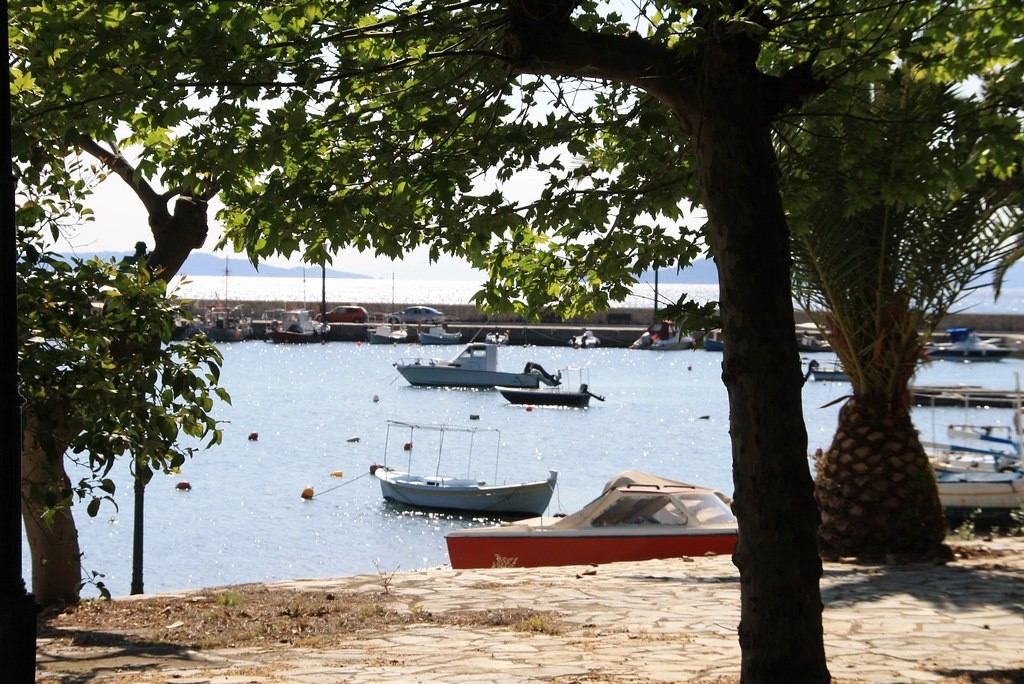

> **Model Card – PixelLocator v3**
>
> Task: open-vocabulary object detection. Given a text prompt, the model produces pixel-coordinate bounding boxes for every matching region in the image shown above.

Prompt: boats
[919,425,1024,510]
[486,330,509,344]
[703,330,725,351]
[376,419,560,516]
[493,386,606,410]
[628,320,695,350]
[263,309,331,342]
[394,344,564,391]
[417,325,460,344]
[795,321,833,351]
[814,368,853,379]
[912,381,1024,410]
[925,329,1008,363]
[571,331,600,347]
[443,468,738,568]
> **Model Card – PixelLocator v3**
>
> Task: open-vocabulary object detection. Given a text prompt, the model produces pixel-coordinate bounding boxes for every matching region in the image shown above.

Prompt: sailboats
[203,253,251,342]
[365,270,409,346]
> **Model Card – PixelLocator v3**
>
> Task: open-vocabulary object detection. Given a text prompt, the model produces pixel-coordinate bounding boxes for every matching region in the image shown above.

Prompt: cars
[313,307,369,324]
[387,306,444,324]
